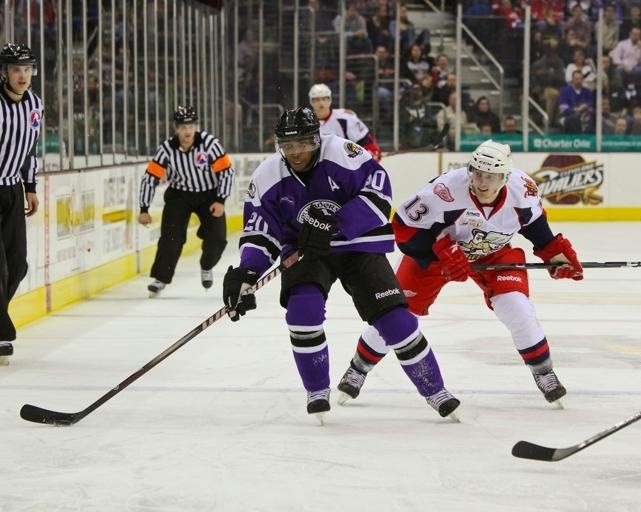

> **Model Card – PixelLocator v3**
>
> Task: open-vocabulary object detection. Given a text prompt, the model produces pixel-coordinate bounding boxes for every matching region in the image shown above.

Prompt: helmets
[468,139,512,183]
[0,43,38,76]
[309,83,332,105]
[173,106,198,124]
[274,106,320,155]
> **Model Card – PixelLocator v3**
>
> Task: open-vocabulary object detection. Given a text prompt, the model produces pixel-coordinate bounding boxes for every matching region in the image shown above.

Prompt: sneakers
[201,270,212,288]
[338,366,365,398]
[534,369,566,403]
[425,387,459,417]
[307,387,330,413]
[148,279,165,291]
[0,341,13,356]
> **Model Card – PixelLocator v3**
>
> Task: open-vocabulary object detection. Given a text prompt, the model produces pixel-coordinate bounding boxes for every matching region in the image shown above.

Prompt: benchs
[133,0,560,152]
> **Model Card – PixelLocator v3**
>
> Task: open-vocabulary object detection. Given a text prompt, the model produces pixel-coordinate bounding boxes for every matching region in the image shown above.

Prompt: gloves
[223,265,258,321]
[296,205,335,260]
[533,233,583,280]
[429,234,469,281]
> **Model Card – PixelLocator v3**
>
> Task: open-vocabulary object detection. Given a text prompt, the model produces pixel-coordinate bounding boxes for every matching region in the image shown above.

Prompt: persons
[136,102,236,294]
[224,1,522,152]
[333,137,586,402]
[421,0,639,134]
[220,104,460,417]
[1,3,229,162]
[0,41,44,356]
